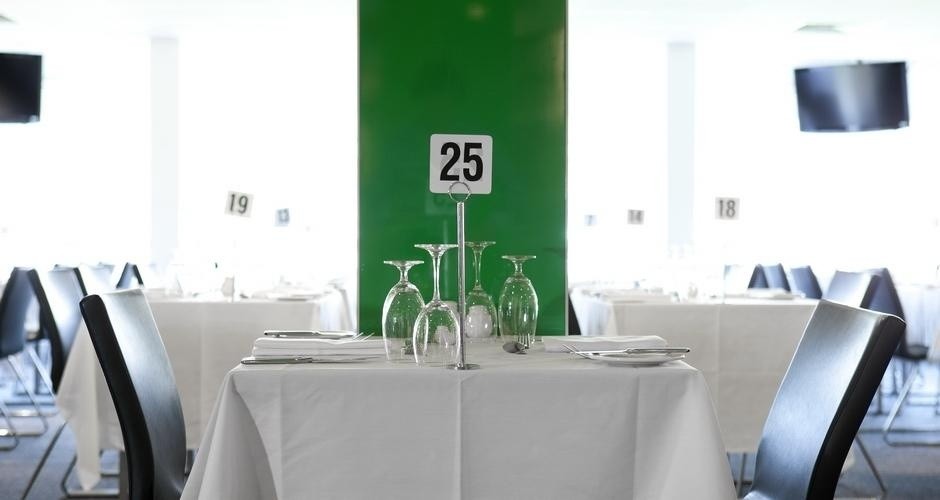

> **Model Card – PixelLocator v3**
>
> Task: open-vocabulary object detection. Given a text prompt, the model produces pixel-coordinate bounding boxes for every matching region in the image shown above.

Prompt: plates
[586,353,685,365]
[271,327,355,339]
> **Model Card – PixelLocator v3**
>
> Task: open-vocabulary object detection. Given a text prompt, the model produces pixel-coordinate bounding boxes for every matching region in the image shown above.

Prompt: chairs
[823,270,883,309]
[5,263,57,419]
[763,264,790,290]
[746,264,767,289]
[18,268,121,500]
[1,267,48,455]
[791,266,823,301]
[724,299,908,499]
[868,265,931,441]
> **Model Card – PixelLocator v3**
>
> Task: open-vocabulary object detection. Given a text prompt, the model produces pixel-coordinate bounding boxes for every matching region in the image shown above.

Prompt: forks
[516,332,535,348]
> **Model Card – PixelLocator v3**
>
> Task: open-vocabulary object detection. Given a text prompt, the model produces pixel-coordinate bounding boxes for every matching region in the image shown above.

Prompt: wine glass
[497,255,537,346]
[381,260,426,363]
[414,244,461,370]
[464,241,498,344]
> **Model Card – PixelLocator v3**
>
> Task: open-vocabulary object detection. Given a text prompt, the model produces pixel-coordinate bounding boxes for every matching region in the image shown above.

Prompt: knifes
[263,330,355,337]
[241,354,380,365]
[569,346,690,355]
